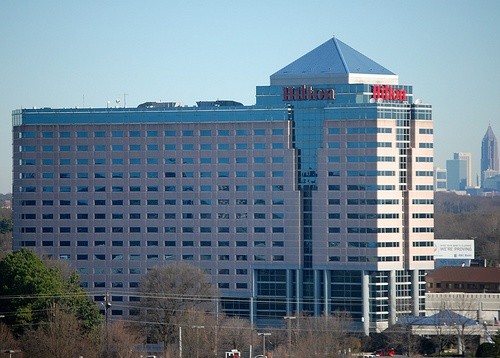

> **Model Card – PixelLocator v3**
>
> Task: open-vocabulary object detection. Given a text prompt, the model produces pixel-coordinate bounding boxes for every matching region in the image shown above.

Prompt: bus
[375,348,395,357]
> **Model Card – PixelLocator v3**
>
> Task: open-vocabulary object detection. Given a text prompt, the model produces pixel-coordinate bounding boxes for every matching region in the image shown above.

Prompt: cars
[440,346,459,355]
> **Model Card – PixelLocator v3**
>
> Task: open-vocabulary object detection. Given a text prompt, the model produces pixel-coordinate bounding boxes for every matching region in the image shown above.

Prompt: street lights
[284,316,297,358]
[258,333,272,358]
[192,325,205,358]
